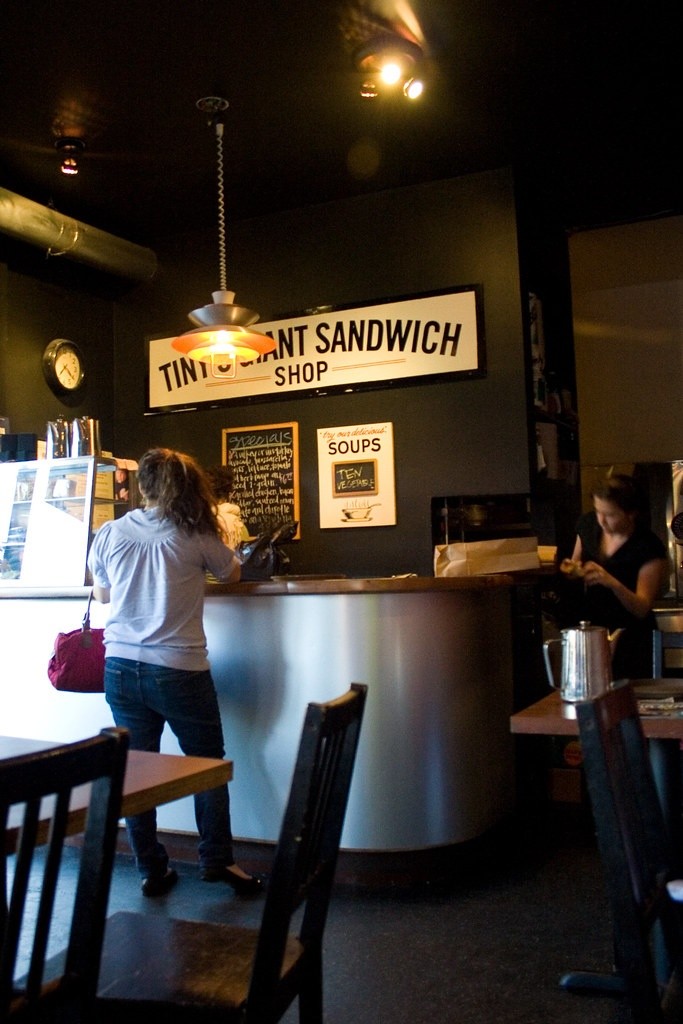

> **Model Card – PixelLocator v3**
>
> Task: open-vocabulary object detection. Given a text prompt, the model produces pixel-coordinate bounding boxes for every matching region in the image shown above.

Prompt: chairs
[14,683,369,1024]
[0,726,131,1024]
[652,631,682,679]
[576,682,683,1024]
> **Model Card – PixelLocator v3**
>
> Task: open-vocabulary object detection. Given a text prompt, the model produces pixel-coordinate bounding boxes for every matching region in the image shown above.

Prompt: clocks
[43,338,86,396]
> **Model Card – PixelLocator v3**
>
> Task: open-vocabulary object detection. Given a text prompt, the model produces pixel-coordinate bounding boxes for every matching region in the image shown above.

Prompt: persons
[197,460,252,584]
[85,450,269,897]
[552,477,665,679]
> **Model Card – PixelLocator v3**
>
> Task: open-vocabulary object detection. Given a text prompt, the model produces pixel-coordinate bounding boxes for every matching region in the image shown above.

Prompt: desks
[0,749,233,855]
[510,678,683,994]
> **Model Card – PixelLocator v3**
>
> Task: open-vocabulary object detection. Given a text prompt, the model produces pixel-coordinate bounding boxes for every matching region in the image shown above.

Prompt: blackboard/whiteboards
[222,420,298,541]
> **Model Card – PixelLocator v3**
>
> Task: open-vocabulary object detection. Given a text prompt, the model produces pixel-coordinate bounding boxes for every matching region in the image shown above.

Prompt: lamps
[172,96,277,378]
[351,37,427,101]
[55,138,85,175]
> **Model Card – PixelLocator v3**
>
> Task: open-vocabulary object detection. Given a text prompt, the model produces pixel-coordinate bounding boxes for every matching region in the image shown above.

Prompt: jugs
[543,620,626,720]
[71,415,103,457]
[46,418,70,459]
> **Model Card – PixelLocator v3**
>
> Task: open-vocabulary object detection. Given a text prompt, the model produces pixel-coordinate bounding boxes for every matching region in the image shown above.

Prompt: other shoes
[140,865,178,898]
[198,864,268,895]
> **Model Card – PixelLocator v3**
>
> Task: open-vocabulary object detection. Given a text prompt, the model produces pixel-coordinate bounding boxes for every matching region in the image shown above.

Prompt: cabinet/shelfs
[0,455,140,588]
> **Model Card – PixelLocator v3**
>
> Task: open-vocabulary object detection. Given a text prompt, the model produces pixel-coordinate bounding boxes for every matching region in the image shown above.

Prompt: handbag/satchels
[433,534,560,578]
[47,588,105,692]
